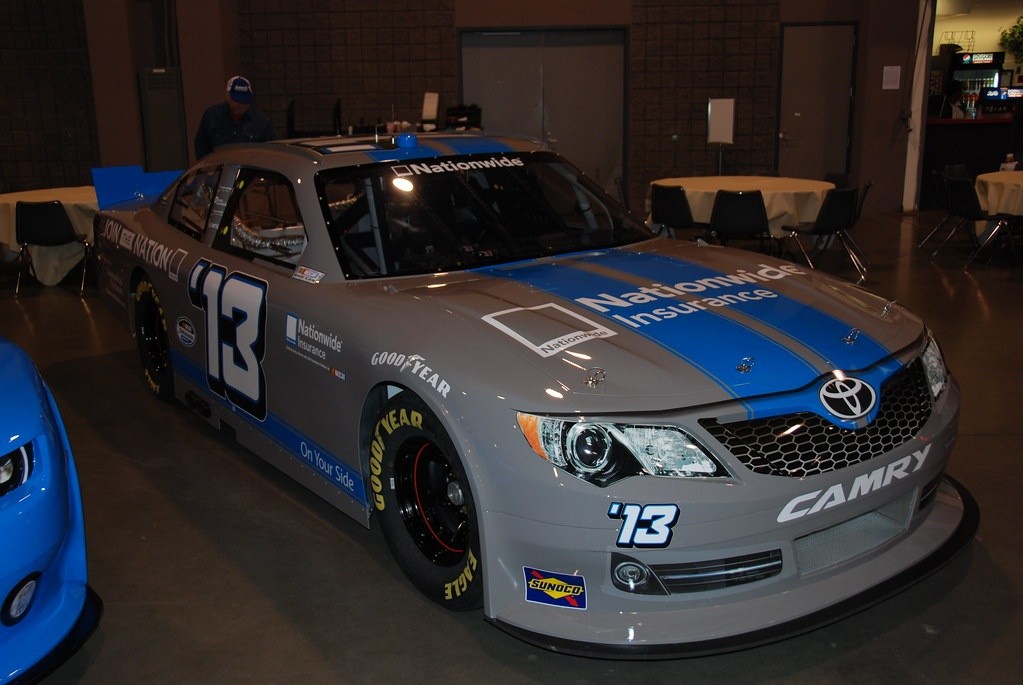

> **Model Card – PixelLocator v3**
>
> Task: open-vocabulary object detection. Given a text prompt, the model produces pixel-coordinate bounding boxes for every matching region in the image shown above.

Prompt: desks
[975,170,1023,267]
[650,176,837,263]
[0,184,100,287]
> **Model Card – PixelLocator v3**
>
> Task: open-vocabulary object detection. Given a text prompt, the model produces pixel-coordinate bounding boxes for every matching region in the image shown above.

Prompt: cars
[0,335,90,685]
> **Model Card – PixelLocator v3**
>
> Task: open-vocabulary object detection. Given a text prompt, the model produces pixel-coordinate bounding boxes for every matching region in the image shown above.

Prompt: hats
[226,76,253,102]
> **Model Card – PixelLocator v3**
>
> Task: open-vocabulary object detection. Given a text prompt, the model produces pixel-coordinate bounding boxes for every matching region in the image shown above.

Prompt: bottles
[1005,153,1014,173]
[957,77,994,108]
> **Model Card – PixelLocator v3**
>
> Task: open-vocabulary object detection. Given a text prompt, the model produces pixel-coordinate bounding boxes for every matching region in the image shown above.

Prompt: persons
[194,74,273,161]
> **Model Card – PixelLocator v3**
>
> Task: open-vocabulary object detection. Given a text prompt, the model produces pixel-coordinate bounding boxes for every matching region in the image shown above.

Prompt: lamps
[707,98,736,176]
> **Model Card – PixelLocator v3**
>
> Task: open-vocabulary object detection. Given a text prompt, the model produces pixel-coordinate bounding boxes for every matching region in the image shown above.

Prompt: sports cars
[87,131,980,661]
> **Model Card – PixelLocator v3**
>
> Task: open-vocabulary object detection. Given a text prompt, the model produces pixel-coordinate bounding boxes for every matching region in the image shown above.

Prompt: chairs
[915,152,1023,271]
[12,200,100,300]
[613,171,874,284]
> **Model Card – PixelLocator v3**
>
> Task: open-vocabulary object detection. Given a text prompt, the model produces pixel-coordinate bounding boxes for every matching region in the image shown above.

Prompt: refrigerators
[945,50,1006,118]
